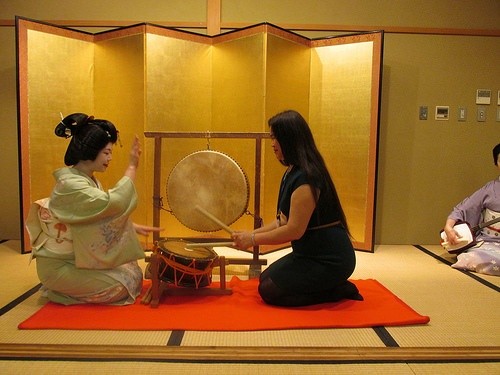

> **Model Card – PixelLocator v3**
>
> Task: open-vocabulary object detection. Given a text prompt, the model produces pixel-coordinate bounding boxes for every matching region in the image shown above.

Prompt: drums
[145,239,217,288]
[166,149,250,232]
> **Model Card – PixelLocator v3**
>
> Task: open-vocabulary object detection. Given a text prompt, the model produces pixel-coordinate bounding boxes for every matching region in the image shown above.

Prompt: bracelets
[250,233,256,247]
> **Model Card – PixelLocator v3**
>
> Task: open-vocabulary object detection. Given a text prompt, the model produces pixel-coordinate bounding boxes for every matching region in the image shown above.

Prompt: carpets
[15,275,431,332]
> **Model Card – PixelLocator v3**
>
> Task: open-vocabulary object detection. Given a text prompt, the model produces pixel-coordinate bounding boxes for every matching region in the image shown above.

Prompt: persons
[444,143,500,275]
[231,110,364,307]
[26,113,166,305]
